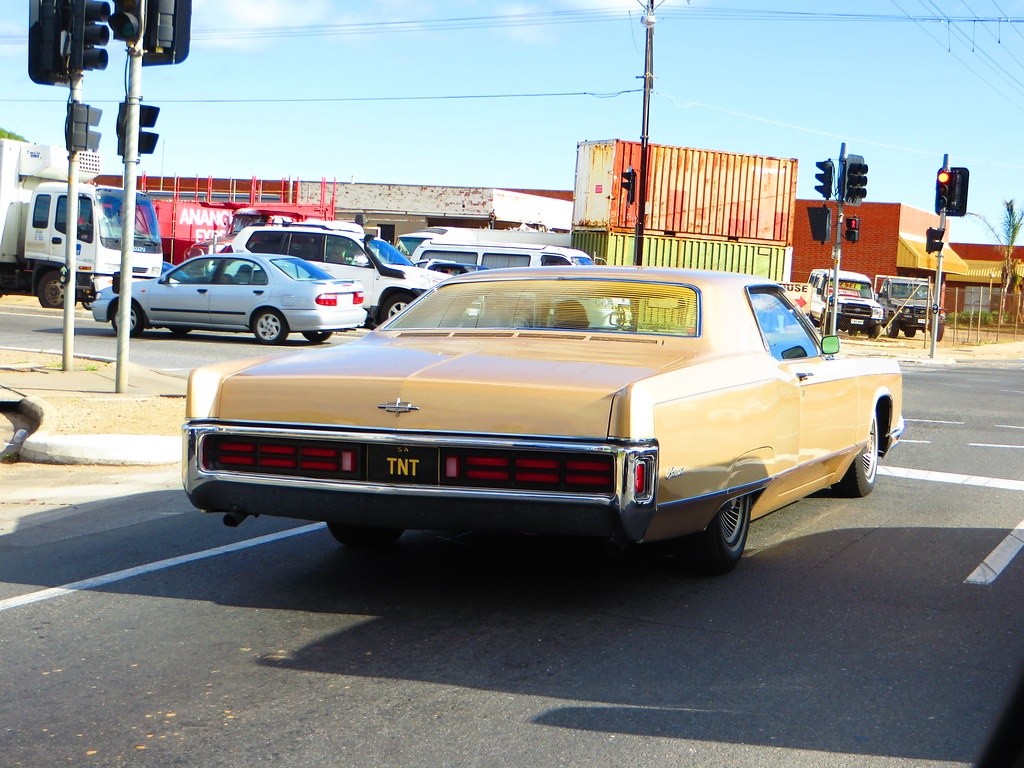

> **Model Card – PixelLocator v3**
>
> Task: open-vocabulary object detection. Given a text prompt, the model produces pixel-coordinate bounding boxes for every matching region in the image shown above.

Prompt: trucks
[0,139,334,310]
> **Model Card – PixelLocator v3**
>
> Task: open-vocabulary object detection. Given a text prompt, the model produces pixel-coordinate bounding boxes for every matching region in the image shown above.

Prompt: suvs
[876,278,947,342]
[211,223,453,329]
[808,269,885,339]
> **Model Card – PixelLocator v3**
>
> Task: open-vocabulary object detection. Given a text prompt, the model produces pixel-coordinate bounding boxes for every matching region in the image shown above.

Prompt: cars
[161,261,189,282]
[92,254,368,346]
[182,265,904,575]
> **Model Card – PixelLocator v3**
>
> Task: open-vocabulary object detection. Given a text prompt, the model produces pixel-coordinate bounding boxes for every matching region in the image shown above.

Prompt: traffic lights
[621,168,635,205]
[935,167,970,217]
[71,0,111,70]
[117,101,160,156]
[108,0,143,43]
[66,100,103,152]
[814,161,833,198]
[844,218,860,242]
[807,206,830,242]
[845,154,869,206]
[925,227,945,254]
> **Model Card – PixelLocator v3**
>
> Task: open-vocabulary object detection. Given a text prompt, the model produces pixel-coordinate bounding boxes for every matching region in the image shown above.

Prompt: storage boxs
[570,230,787,330]
[573,139,798,247]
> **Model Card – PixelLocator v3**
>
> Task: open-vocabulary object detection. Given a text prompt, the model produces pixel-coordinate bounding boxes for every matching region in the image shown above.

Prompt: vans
[397,225,617,331]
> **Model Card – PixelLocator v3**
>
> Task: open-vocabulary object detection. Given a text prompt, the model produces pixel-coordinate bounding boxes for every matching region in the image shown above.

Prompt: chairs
[235,264,253,285]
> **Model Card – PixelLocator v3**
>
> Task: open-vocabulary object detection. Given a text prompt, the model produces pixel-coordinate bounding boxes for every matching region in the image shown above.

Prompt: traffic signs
[778,283,812,315]
